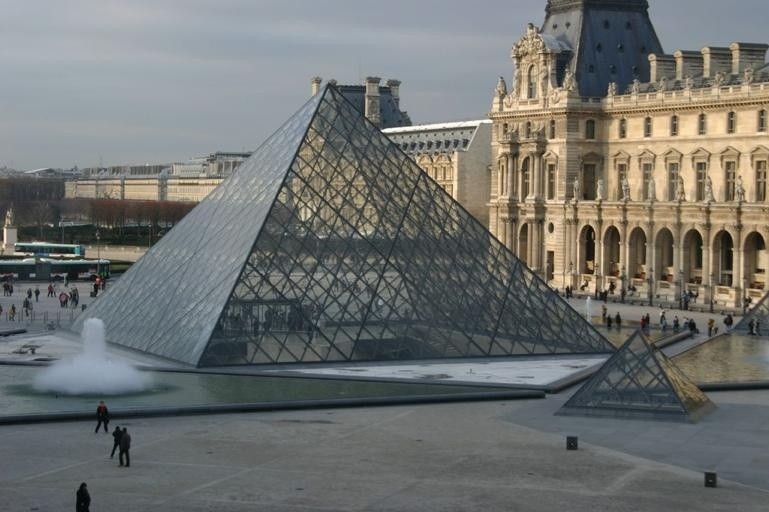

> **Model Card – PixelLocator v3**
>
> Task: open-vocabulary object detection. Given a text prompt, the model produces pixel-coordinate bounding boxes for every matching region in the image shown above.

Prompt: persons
[0,274,106,321]
[95,400,110,433]
[217,300,315,331]
[110,425,131,467]
[333,277,362,294]
[76,483,91,512]
[553,279,754,337]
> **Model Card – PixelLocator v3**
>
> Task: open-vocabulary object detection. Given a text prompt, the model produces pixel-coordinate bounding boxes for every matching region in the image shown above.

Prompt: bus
[43,218,171,239]
[13,242,85,258]
[0,259,111,282]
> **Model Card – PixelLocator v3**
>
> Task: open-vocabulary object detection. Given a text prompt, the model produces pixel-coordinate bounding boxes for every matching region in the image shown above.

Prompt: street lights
[95,229,102,277]
[567,260,574,293]
[147,219,152,250]
[648,266,655,304]
[742,275,749,315]
[592,263,600,299]
[546,260,551,286]
[620,264,628,305]
[677,268,685,310]
[708,271,715,313]
